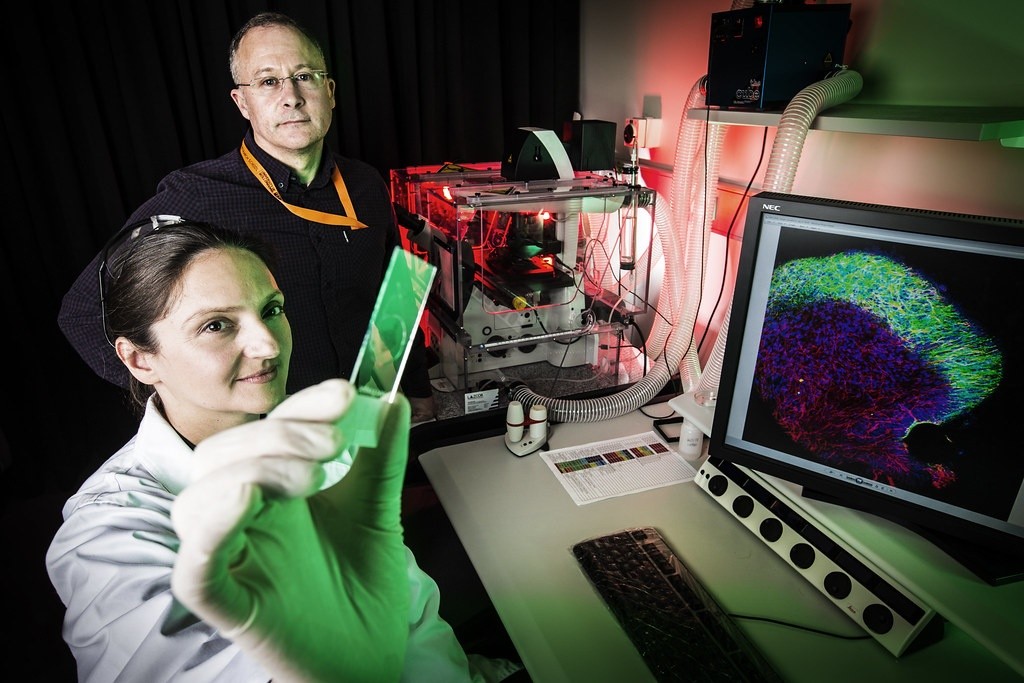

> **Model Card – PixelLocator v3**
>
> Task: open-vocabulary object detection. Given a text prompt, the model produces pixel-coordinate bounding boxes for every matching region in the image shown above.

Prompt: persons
[54,10,436,429]
[44,220,536,683]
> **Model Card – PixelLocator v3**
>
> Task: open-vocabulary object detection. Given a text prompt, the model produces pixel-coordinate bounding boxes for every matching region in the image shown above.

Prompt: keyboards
[572,526,789,683]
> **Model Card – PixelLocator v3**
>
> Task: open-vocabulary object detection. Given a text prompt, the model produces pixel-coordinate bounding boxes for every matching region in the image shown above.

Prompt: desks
[415,398,1021,683]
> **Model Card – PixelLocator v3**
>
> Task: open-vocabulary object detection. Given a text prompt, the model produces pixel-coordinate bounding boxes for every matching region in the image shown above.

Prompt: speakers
[705,7,821,109]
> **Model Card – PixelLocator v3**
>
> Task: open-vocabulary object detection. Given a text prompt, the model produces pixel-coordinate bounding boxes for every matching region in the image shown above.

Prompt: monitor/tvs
[708,190,1024,562]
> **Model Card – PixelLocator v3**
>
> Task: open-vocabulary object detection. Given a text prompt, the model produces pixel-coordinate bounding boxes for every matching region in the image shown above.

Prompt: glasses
[236,70,328,94]
